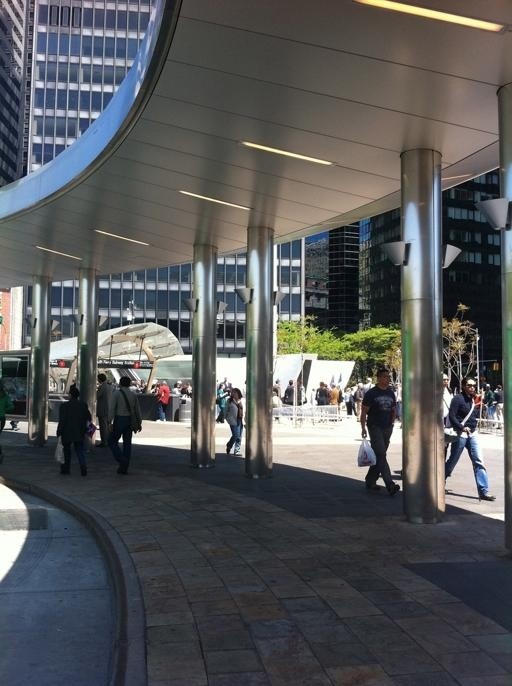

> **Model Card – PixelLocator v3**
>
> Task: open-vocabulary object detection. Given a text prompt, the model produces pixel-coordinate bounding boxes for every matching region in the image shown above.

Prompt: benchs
[474,417,503,438]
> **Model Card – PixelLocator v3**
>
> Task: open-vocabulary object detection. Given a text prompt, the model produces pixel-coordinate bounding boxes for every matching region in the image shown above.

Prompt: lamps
[99,314,109,327]
[474,197,512,231]
[51,319,60,332]
[274,290,287,306]
[217,301,229,315]
[441,244,462,269]
[23,316,36,328]
[234,288,251,304]
[380,241,406,267]
[69,313,83,327]
[181,298,198,313]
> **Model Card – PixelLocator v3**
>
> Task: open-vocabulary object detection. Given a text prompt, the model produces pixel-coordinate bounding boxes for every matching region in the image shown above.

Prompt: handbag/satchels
[444,428,459,443]
[130,412,139,433]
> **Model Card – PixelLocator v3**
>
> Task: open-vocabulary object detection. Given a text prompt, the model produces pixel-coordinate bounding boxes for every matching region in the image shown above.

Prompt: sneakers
[60,464,128,477]
[480,494,496,501]
[226,443,242,457]
[365,480,400,496]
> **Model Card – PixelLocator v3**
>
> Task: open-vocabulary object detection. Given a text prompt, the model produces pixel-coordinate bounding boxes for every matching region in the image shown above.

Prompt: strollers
[471,401,489,429]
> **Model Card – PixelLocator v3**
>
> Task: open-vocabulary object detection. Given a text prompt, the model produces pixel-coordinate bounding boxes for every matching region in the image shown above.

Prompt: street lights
[126,298,138,324]
[459,325,481,395]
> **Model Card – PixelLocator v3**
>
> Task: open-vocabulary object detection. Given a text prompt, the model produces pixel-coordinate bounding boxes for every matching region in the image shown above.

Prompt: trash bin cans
[179,398,191,423]
